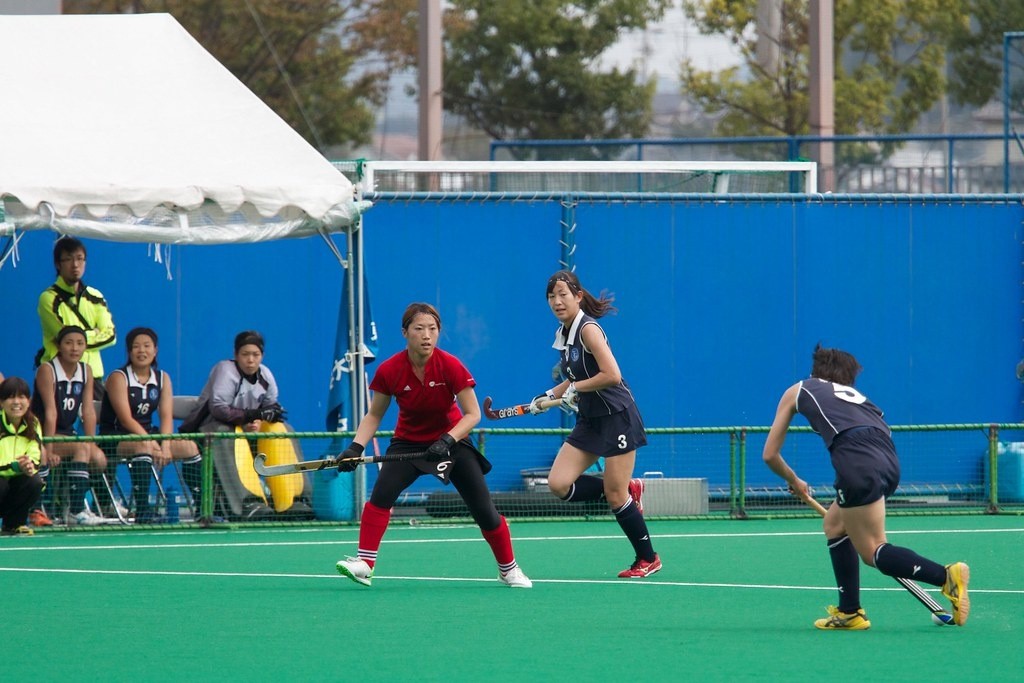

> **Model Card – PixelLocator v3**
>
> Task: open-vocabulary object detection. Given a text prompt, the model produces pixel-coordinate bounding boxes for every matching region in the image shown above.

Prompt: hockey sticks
[483,393,582,421]
[253,449,451,477]
[788,485,956,625]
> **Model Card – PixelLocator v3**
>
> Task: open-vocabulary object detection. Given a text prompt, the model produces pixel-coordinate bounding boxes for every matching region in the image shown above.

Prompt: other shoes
[136,510,178,524]
[195,513,222,523]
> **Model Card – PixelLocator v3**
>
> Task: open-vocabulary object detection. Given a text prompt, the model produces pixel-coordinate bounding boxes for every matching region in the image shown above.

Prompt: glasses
[60,256,86,264]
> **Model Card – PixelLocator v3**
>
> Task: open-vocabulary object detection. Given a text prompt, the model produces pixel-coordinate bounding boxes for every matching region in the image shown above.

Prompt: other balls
[931,613,945,626]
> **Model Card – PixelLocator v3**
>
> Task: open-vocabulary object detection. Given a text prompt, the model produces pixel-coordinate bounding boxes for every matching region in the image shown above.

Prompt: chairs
[41,396,229,525]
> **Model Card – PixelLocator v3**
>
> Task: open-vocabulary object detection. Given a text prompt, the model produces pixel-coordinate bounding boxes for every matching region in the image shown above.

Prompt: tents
[0,12,376,534]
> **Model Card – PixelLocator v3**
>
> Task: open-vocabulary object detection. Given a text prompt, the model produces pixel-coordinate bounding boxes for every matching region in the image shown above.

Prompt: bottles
[165,484,179,522]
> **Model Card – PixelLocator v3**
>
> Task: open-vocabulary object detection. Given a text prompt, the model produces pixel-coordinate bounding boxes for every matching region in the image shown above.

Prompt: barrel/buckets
[983,442,1024,502]
[306,468,355,522]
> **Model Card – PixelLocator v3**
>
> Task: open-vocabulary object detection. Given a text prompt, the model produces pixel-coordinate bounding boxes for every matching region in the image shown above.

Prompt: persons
[38,238,117,400]
[530,271,662,579]
[0,376,42,534]
[178,331,289,517]
[763,338,971,631]
[32,325,107,527]
[336,302,533,588]
[99,327,231,524]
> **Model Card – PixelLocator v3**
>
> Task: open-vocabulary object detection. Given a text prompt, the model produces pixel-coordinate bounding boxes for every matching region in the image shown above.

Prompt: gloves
[333,443,365,472]
[426,433,457,461]
[529,389,557,414]
[259,403,289,424]
[562,382,580,414]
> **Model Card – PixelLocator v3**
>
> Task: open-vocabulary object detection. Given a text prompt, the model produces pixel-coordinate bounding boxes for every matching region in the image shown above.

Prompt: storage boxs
[984,441,1024,503]
[313,467,367,521]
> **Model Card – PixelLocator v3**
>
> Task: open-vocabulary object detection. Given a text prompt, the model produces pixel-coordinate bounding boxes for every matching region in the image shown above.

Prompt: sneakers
[499,568,533,588]
[940,562,970,627]
[337,553,375,587]
[618,552,663,578]
[68,509,107,526]
[629,479,644,516]
[813,606,870,630]
[108,502,128,515]
[30,508,54,527]
